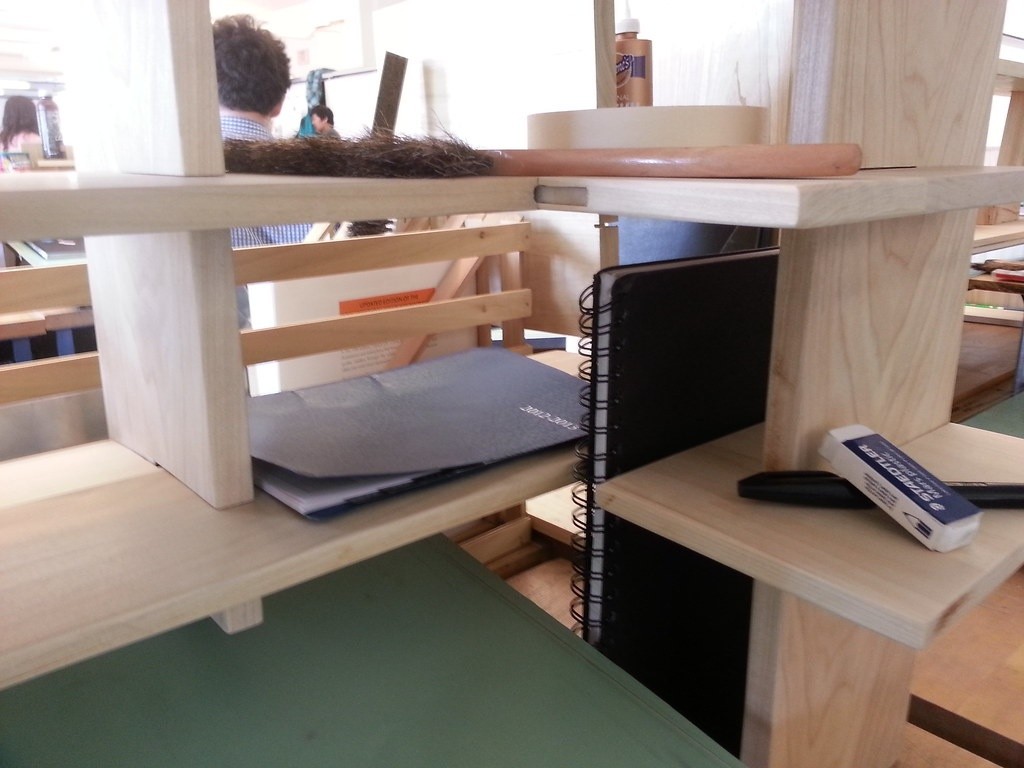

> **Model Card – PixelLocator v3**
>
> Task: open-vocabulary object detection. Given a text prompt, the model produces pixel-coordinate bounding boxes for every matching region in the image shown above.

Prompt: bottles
[36,89,67,161]
[614,18,654,108]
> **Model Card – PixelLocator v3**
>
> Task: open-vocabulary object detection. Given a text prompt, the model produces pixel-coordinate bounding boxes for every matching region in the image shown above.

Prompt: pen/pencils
[738,470,1023,509]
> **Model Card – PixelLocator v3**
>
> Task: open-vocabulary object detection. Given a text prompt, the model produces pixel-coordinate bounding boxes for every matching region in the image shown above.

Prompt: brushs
[223,127,860,179]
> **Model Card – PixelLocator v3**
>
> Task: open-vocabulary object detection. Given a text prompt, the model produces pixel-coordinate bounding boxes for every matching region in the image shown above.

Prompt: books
[569,245,784,768]
[248,343,593,524]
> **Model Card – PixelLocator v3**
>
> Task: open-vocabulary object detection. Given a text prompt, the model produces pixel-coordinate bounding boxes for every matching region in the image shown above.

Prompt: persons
[210,15,314,329]
[309,105,340,138]
[0,94,48,170]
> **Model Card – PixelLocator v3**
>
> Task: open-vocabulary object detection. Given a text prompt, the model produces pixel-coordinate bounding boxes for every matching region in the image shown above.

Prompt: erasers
[816,423,982,552]
